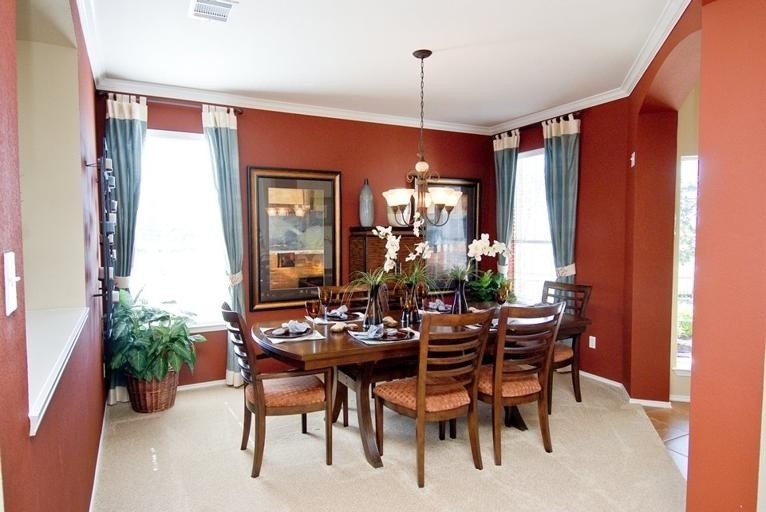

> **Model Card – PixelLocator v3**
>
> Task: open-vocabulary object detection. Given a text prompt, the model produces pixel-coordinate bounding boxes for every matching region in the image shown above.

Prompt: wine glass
[316,285,332,320]
[305,297,322,335]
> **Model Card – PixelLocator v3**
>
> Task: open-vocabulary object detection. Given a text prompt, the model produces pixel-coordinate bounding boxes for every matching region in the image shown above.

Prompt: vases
[434,260,488,315]
[336,263,402,330]
[388,247,445,327]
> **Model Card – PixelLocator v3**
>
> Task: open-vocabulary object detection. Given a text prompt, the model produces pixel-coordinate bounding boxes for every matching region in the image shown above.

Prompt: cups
[495,280,512,309]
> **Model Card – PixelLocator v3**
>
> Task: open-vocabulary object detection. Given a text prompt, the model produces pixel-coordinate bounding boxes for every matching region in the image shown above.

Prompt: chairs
[375,280,432,312]
[371,305,497,489]
[448,299,568,466]
[317,280,374,313]
[220,301,333,480]
[528,279,594,416]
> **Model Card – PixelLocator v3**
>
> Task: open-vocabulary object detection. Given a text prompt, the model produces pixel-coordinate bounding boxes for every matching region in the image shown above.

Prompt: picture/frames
[247,166,351,313]
[408,172,485,290]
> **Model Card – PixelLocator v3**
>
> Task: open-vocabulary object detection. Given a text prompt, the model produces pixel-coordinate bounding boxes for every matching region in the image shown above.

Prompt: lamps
[381,47,463,235]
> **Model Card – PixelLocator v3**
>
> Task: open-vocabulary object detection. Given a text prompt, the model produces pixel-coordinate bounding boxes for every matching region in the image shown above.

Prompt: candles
[96,157,119,281]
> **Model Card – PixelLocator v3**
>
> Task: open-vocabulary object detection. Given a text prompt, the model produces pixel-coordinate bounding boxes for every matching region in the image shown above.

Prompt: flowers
[337,212,518,315]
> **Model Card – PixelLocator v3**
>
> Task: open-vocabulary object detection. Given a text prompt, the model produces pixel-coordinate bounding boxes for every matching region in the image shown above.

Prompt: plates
[373,329,416,341]
[317,313,359,322]
[262,326,311,338]
[425,303,504,330]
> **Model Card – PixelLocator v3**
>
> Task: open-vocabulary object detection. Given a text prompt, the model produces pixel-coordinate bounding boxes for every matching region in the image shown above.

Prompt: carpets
[89,361,688,511]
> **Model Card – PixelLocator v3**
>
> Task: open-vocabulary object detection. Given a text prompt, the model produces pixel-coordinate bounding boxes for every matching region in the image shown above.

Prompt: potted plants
[104,287,209,415]
